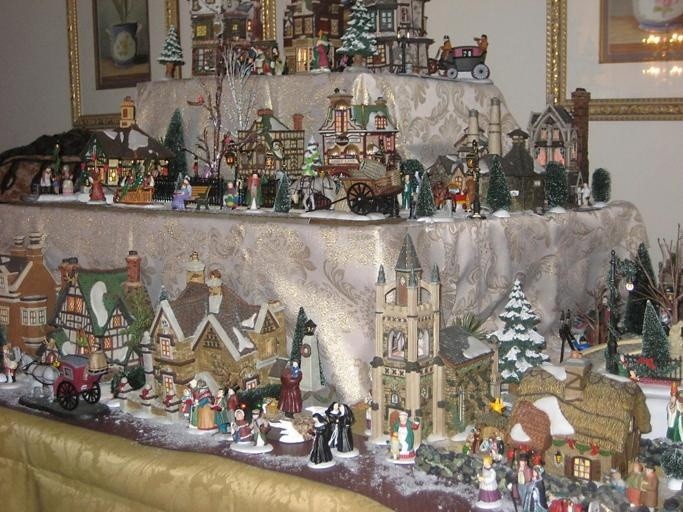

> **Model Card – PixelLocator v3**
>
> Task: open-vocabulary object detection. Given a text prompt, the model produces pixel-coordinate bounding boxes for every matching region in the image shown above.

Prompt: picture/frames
[63,2,179,138]
[544,2,683,124]
